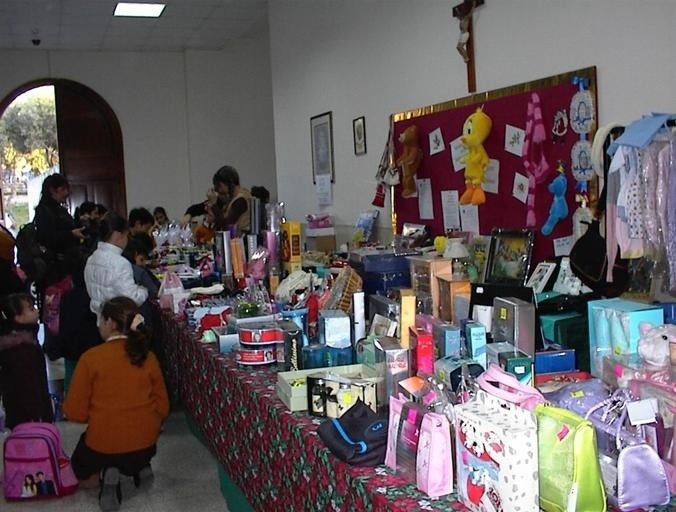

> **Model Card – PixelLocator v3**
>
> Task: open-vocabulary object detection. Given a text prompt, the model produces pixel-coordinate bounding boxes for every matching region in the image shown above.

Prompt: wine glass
[152,221,193,255]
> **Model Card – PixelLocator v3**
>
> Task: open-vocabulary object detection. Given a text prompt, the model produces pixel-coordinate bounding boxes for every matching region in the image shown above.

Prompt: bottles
[214,199,285,318]
[412,267,434,315]
[309,380,351,418]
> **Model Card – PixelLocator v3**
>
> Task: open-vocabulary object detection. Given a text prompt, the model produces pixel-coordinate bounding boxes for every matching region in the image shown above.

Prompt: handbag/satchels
[317,395,389,466]
[566,219,633,298]
[385,362,676,512]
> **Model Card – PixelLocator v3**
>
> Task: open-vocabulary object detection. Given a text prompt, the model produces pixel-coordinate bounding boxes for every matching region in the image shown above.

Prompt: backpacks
[15,221,50,282]
[42,274,75,335]
[0,420,82,504]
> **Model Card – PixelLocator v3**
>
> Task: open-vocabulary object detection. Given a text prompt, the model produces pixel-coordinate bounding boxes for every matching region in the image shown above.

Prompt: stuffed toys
[542,173,569,236]
[396,125,422,198]
[459,108,493,205]
[615,321,676,389]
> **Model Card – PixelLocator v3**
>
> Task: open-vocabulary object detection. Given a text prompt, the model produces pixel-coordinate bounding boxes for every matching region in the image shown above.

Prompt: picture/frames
[479,226,535,287]
[309,110,336,186]
[351,116,368,157]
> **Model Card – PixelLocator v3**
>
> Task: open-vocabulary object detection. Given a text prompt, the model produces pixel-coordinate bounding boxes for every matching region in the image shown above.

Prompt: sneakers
[95,463,122,512]
[133,463,154,491]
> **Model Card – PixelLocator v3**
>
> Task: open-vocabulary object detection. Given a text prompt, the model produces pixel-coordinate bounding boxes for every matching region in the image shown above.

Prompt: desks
[146,291,675,512]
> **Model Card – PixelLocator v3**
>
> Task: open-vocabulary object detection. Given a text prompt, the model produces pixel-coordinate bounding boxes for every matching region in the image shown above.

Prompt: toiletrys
[337,384,352,418]
[396,409,420,485]
[311,379,326,418]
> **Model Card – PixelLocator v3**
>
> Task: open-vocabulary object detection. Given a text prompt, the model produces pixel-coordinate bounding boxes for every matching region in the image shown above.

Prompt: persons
[456,1,476,62]
